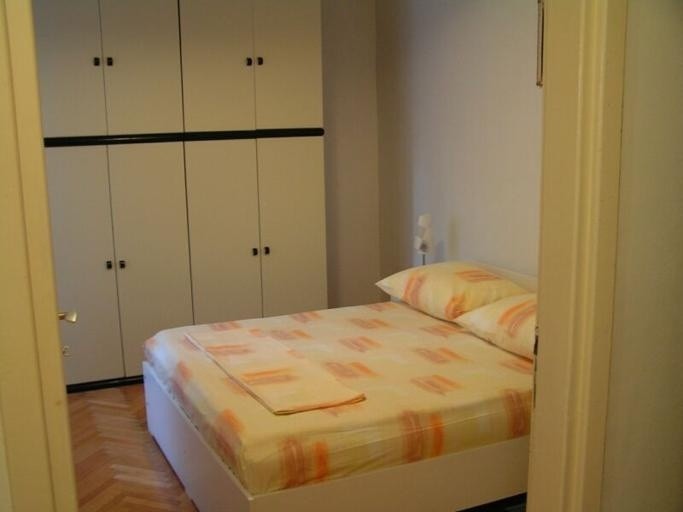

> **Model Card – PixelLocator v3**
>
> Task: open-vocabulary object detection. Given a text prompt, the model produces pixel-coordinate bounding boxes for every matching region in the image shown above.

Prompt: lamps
[412,213,434,264]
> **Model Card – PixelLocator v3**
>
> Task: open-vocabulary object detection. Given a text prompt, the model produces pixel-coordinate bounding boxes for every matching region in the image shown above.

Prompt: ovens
[141,260,536,511]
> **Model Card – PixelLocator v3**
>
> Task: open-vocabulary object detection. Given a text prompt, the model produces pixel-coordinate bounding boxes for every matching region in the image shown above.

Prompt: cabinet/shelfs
[184,132,327,324]
[43,138,195,388]
[32,1,183,141]
[177,0,325,132]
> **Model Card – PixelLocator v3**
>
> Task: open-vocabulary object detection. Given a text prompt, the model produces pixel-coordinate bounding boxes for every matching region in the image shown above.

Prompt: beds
[141,260,536,511]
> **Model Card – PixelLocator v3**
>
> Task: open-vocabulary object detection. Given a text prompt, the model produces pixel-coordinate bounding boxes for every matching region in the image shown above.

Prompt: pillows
[375,262,536,361]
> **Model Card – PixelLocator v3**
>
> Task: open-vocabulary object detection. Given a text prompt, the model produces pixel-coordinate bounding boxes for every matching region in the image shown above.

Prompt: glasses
[412,213,434,264]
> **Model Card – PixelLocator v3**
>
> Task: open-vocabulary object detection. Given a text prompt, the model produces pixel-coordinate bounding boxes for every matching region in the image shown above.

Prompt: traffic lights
[375,262,536,361]
